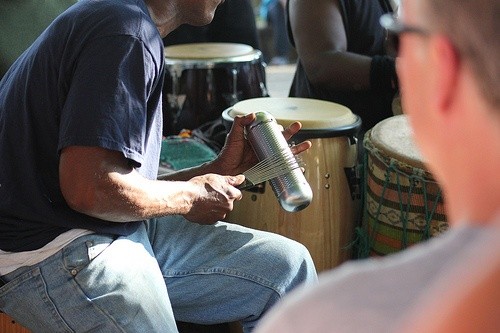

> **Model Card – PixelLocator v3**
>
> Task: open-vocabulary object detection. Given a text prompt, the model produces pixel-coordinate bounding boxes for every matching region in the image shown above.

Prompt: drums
[163,42,268,138]
[222,98,361,278]
[362,114,447,257]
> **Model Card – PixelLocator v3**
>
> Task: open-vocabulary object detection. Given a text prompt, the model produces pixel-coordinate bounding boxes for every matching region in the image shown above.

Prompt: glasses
[379,13,429,56]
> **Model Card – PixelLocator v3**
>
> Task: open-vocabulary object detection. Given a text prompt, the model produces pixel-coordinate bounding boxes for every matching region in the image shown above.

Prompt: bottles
[244,111,313,212]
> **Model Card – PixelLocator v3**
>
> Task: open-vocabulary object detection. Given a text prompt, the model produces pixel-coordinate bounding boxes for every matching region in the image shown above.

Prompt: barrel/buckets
[160,42,270,141]
[210,97,361,274]
[364,114,448,254]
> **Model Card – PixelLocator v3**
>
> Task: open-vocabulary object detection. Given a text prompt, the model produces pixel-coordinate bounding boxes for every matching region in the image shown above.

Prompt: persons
[1,0,319,333]
[284,0,404,220]
[250,0,500,333]
